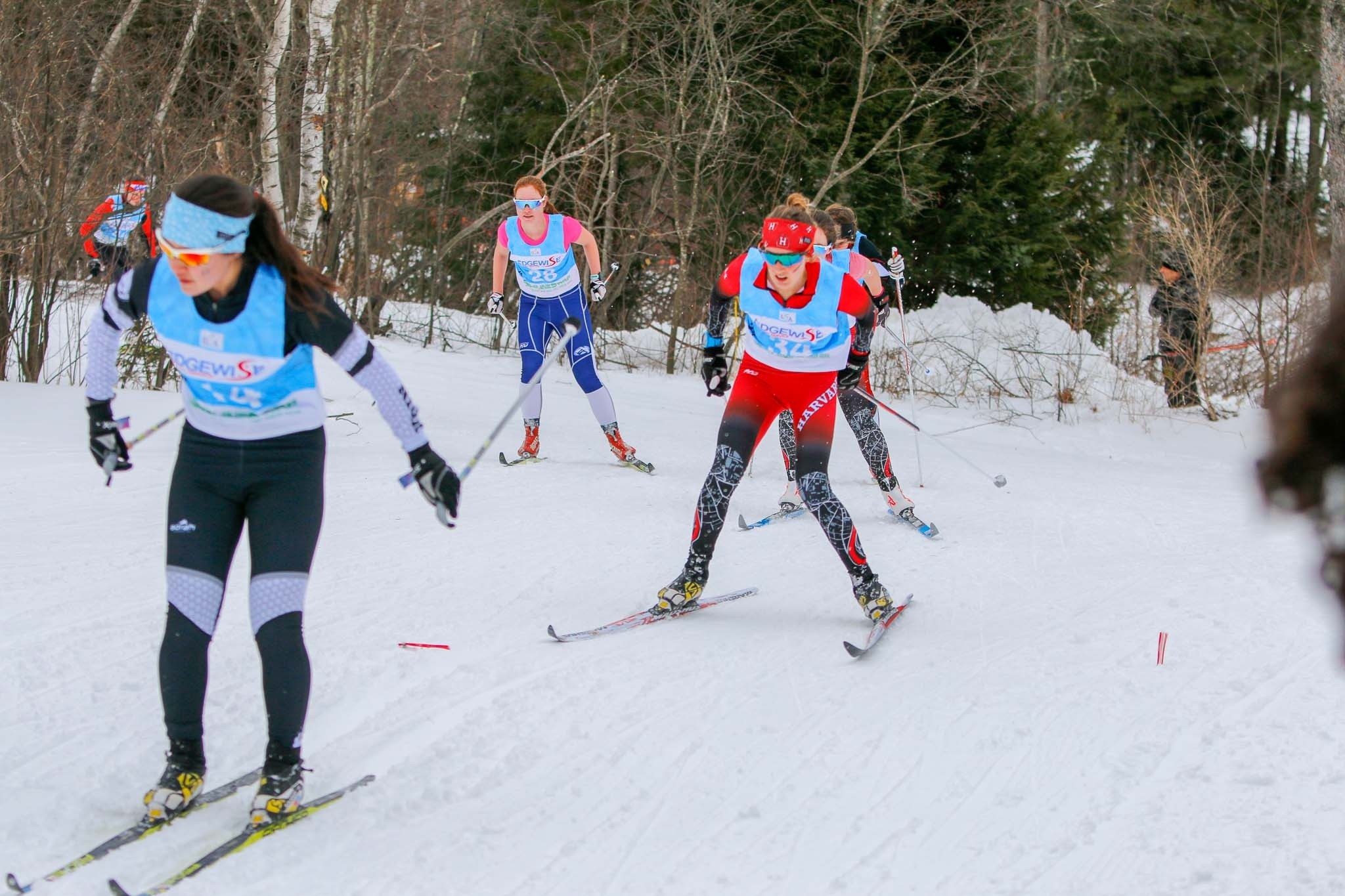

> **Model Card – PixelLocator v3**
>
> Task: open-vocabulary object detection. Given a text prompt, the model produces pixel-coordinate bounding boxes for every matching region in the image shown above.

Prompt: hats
[1163,251,1188,273]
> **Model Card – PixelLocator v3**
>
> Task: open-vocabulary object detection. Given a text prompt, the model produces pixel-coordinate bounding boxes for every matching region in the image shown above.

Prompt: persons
[85,174,459,832]
[1149,245,1212,409]
[825,202,906,484]
[487,175,635,464]
[777,204,916,515]
[80,174,158,284]
[656,192,893,625]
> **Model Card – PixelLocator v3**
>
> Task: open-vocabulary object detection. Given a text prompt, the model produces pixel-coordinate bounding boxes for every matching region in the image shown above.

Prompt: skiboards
[738,506,940,540]
[498,451,655,474]
[4,767,377,895]
[548,587,914,658]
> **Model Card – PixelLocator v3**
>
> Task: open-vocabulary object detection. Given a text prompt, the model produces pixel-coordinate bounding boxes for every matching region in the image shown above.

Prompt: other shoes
[142,749,206,822]
[879,476,915,516]
[778,469,804,506]
[853,573,893,622]
[656,567,709,610]
[600,421,636,460]
[517,418,540,457]
[251,740,305,829]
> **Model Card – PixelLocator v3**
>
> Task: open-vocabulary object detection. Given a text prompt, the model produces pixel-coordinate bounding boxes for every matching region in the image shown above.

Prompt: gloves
[873,285,890,328]
[837,346,870,392]
[487,292,504,314]
[86,400,132,486]
[399,442,459,518]
[700,342,731,397]
[590,272,607,302]
[887,254,905,278]
[87,258,101,278]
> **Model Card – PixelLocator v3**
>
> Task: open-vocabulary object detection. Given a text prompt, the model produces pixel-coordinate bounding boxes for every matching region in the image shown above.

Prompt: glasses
[513,194,545,210]
[834,234,856,250]
[813,242,833,258]
[757,240,814,267]
[155,226,246,270]
